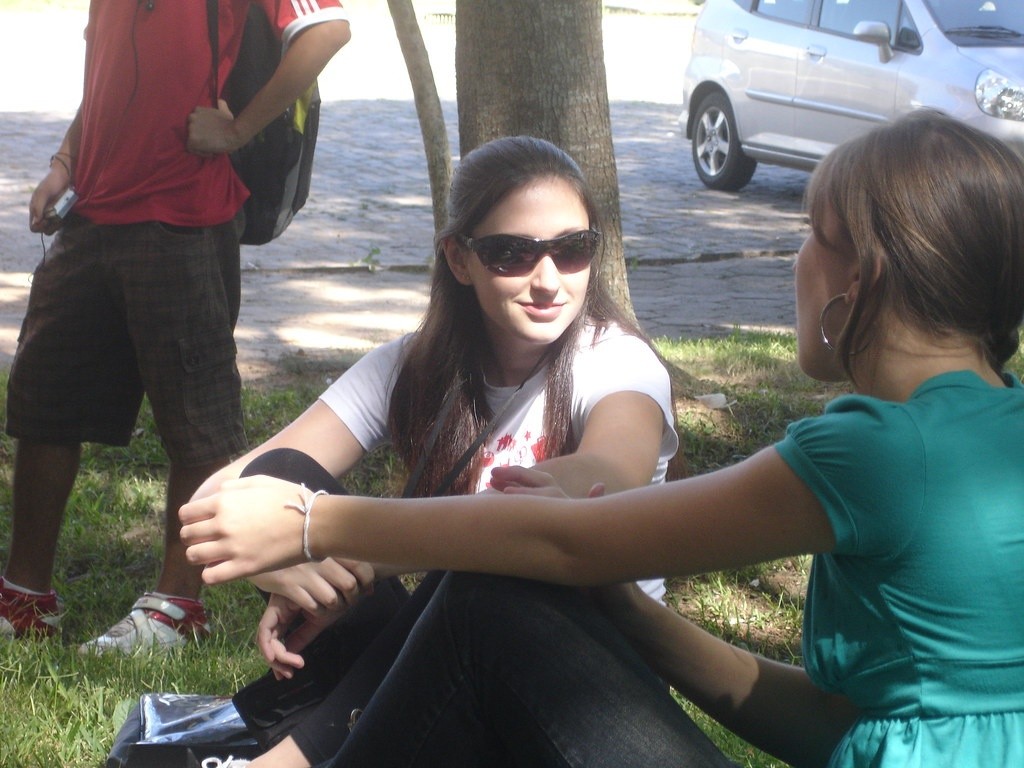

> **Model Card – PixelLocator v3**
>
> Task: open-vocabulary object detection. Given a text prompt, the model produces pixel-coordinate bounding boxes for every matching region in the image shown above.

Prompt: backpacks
[206,0,321,245]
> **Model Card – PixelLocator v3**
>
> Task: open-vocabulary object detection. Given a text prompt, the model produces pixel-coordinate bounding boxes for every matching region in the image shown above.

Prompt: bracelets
[49,154,73,177]
[282,482,330,560]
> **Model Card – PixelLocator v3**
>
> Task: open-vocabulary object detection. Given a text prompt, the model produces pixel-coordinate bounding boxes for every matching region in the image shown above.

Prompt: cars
[678,0,1024,187]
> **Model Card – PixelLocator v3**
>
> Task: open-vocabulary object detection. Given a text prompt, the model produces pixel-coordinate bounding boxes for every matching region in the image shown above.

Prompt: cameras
[42,186,79,225]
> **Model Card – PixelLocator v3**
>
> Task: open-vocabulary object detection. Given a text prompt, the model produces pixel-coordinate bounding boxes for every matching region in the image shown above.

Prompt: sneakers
[79,592,210,660]
[0,574,61,638]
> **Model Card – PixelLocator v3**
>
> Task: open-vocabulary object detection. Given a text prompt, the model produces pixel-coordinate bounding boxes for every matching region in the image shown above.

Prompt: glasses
[456,227,601,277]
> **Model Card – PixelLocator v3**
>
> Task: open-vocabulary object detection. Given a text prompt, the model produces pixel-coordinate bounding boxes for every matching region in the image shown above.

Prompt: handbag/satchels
[106,691,264,768]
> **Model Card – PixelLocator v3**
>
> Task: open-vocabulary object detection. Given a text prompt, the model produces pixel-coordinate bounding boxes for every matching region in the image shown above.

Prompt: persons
[0,0,358,660]
[183,133,689,691]
[197,104,1024,768]
